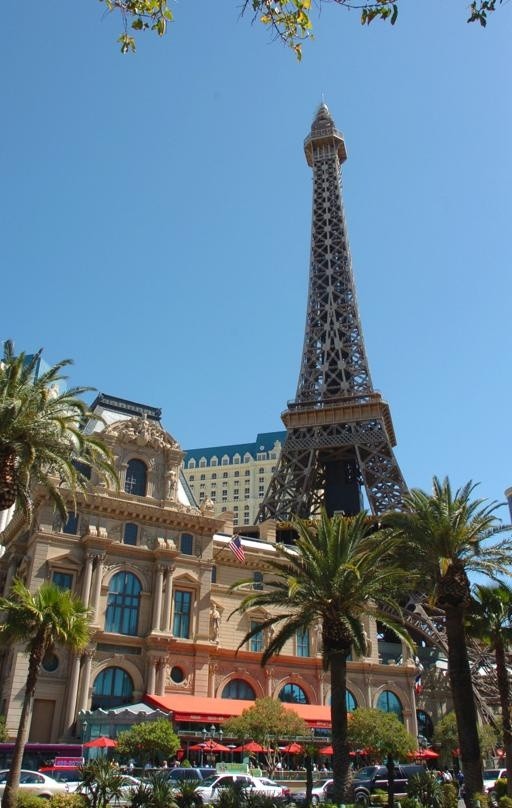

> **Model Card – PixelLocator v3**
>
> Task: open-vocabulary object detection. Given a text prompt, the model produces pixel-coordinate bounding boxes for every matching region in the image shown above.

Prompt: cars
[0,764,290,807]
[481,766,508,793]
[37,755,99,795]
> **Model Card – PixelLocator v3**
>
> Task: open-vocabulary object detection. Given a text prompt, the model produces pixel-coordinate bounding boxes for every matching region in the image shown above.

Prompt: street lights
[417,733,428,766]
[80,717,89,758]
[200,723,224,768]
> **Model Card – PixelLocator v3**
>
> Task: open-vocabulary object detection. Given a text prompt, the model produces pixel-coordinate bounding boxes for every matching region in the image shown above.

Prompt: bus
[0,742,85,774]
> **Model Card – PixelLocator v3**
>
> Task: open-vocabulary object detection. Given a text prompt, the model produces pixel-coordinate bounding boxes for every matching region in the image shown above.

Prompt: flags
[228,536,246,562]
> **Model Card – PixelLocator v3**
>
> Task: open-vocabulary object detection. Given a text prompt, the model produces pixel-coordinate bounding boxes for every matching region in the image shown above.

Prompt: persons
[427,769,465,786]
[209,603,220,642]
[313,617,325,652]
[90,758,216,769]
[249,759,328,779]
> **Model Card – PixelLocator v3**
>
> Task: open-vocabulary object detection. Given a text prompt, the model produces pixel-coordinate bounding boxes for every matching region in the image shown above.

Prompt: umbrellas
[186,737,374,765]
[398,747,441,759]
[83,735,122,763]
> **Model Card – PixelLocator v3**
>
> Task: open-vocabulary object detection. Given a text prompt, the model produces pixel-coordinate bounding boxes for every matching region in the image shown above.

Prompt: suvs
[319,762,433,805]
[290,774,335,806]
